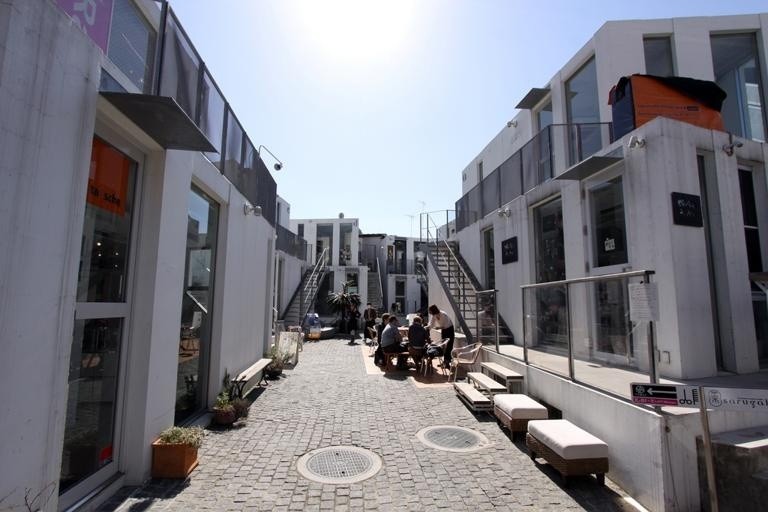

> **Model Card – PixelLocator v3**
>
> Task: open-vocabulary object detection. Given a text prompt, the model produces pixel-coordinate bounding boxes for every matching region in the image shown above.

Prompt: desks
[397,327,410,337]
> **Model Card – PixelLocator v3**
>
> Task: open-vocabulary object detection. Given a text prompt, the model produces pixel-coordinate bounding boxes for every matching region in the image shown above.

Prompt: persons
[362,302,377,340]
[423,304,454,368]
[378,313,390,347]
[408,316,430,367]
[417,313,430,340]
[381,315,409,368]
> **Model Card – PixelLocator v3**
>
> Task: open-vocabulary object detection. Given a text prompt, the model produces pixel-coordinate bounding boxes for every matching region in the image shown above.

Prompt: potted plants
[325,280,362,334]
[151,425,205,479]
[263,346,294,380]
[212,389,248,425]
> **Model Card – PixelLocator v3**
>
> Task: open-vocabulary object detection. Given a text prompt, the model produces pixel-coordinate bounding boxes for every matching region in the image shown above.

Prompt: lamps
[722,140,743,156]
[244,200,263,216]
[507,120,517,127]
[498,209,511,217]
[628,136,646,148]
[255,144,282,170]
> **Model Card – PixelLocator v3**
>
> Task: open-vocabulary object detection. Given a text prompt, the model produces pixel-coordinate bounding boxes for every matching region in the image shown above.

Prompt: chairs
[367,327,380,354]
[420,338,450,377]
[181,311,202,354]
[447,342,483,382]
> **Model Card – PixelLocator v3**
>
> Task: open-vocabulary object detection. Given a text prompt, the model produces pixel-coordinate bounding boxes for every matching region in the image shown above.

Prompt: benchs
[384,352,432,371]
[231,358,273,400]
[525,419,609,487]
[492,392,549,443]
[321,327,337,340]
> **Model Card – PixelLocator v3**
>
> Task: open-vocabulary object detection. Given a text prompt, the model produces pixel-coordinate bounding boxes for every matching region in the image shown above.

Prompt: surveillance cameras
[628,135,637,148]
[734,139,743,147]
[507,121,511,127]
